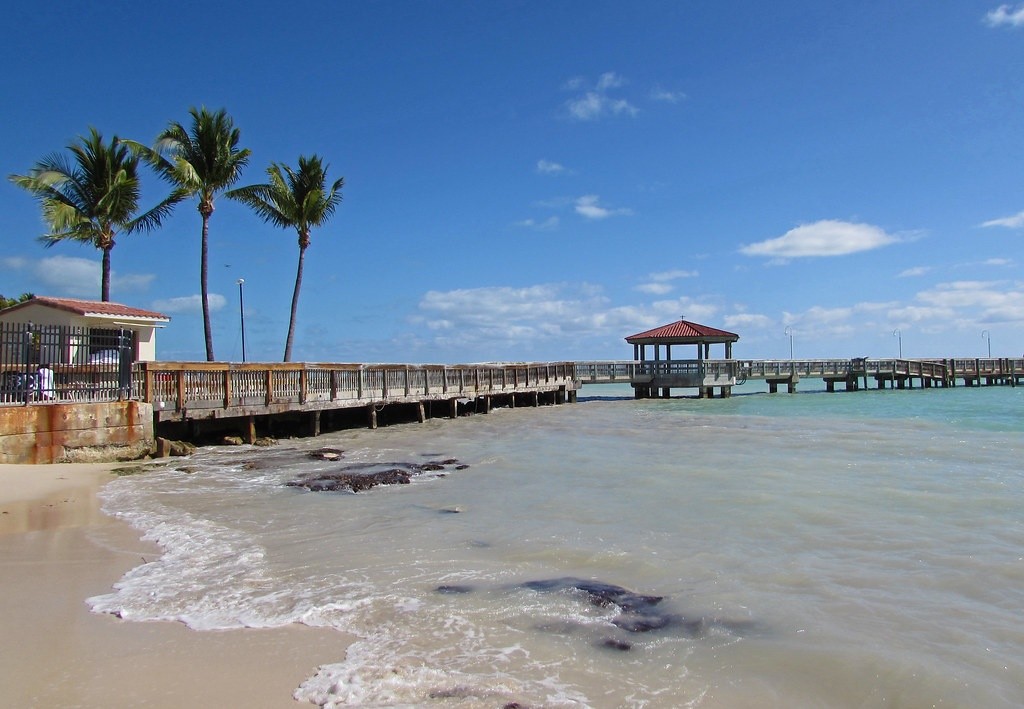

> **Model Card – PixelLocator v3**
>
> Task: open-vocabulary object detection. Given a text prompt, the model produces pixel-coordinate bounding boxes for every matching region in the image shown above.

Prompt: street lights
[238,278,250,362]
[785,325,794,359]
[982,329,991,358]
[893,328,902,359]
